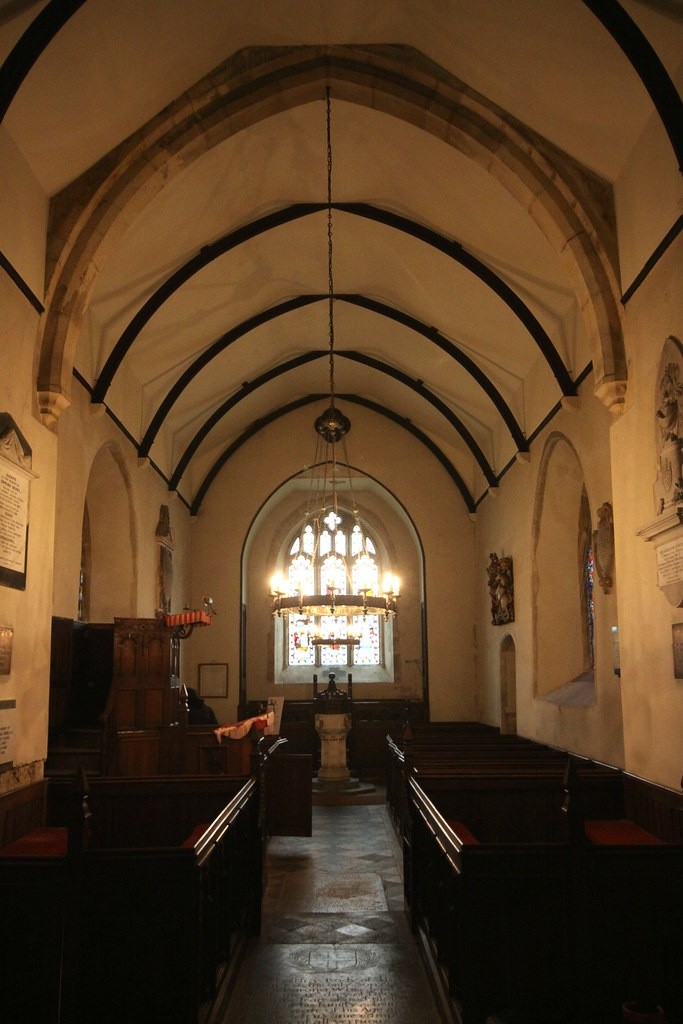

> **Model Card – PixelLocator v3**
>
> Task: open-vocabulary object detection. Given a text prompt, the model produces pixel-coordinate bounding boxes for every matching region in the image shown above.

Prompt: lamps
[263,83,407,652]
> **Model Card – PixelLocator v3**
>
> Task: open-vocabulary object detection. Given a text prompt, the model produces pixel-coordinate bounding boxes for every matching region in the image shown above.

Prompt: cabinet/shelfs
[46,615,251,779]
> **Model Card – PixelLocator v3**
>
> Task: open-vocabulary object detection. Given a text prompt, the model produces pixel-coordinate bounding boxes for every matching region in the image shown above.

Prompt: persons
[187,687,219,725]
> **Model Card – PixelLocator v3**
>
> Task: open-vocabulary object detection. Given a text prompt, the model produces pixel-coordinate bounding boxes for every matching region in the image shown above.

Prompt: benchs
[0,772,265,1024]
[407,762,683,1024]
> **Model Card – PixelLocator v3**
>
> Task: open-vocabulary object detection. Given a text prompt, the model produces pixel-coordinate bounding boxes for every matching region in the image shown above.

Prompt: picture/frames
[196,662,229,700]
